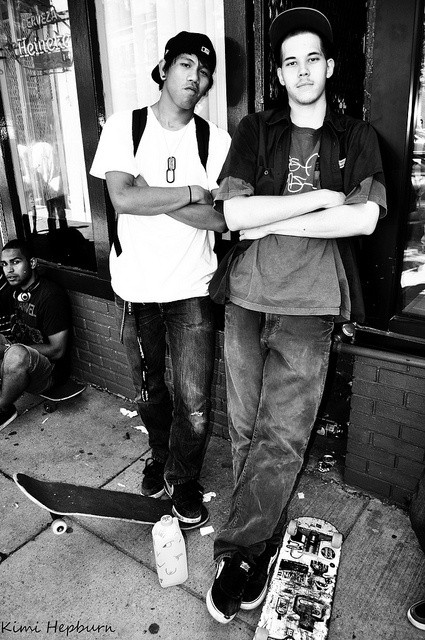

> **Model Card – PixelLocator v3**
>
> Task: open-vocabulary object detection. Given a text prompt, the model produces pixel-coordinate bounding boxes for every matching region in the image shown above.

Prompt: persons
[207,8,388,624]
[0,239,72,430]
[91,31,236,524]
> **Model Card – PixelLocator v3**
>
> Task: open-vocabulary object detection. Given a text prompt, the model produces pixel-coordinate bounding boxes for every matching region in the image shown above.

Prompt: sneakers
[240,545,279,610]
[141,458,165,498]
[0,406,18,431]
[206,557,255,623]
[164,479,204,523]
[405,600,425,630]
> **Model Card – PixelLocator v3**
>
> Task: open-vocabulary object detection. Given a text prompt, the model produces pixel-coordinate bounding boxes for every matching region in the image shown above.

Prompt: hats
[269,8,333,67]
[152,31,216,84]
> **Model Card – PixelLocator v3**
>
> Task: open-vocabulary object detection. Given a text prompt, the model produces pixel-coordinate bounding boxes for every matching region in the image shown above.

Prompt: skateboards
[39,381,86,413]
[12,473,210,536]
[252,517,344,640]
